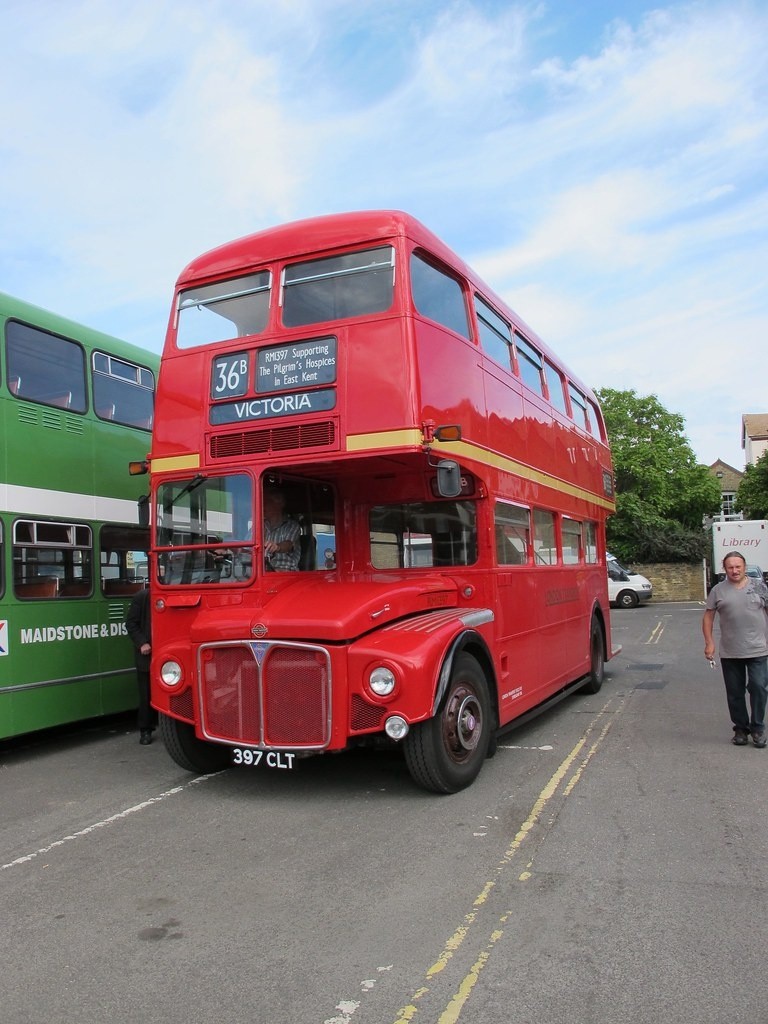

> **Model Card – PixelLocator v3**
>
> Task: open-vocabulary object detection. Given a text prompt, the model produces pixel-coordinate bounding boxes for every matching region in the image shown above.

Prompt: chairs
[290,514,311,570]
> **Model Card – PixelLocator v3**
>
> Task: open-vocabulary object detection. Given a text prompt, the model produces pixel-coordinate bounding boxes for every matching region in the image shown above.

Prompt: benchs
[10,374,152,429]
[13,575,147,598]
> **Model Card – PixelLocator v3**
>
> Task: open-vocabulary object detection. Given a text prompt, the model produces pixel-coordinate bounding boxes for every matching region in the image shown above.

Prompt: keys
[709,657,719,670]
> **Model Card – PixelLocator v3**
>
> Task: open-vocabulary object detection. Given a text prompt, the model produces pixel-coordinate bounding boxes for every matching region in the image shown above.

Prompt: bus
[126,207,617,796]
[1,288,254,741]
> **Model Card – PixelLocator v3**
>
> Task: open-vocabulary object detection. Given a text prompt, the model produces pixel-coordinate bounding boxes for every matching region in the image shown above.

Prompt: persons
[211,487,302,574]
[126,586,158,745]
[702,551,768,749]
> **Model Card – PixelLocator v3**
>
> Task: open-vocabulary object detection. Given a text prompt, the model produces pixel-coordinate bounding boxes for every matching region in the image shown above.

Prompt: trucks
[702,519,768,599]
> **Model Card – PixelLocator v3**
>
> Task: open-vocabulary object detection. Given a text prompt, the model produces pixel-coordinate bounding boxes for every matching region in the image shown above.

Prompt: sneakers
[141,730,152,745]
[731,727,768,746]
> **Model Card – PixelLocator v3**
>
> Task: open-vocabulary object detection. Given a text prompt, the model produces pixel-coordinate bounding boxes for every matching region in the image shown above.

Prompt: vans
[537,545,654,608]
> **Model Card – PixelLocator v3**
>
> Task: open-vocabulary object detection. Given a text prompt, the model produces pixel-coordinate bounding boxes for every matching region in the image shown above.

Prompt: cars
[724,564,763,588]
